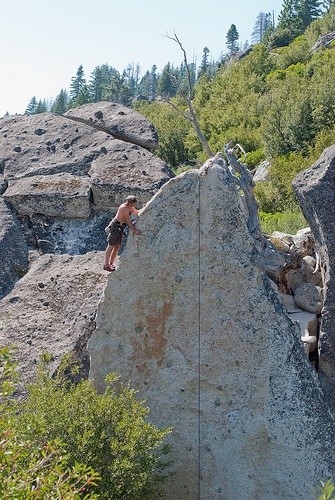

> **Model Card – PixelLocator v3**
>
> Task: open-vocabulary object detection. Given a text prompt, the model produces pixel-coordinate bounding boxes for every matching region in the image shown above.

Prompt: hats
[126,196,137,202]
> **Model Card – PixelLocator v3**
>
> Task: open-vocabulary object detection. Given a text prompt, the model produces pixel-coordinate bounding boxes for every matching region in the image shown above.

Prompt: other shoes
[103,265,116,271]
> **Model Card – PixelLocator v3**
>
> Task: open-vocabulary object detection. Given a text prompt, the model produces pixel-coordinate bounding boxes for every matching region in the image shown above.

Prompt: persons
[103,195,140,272]
